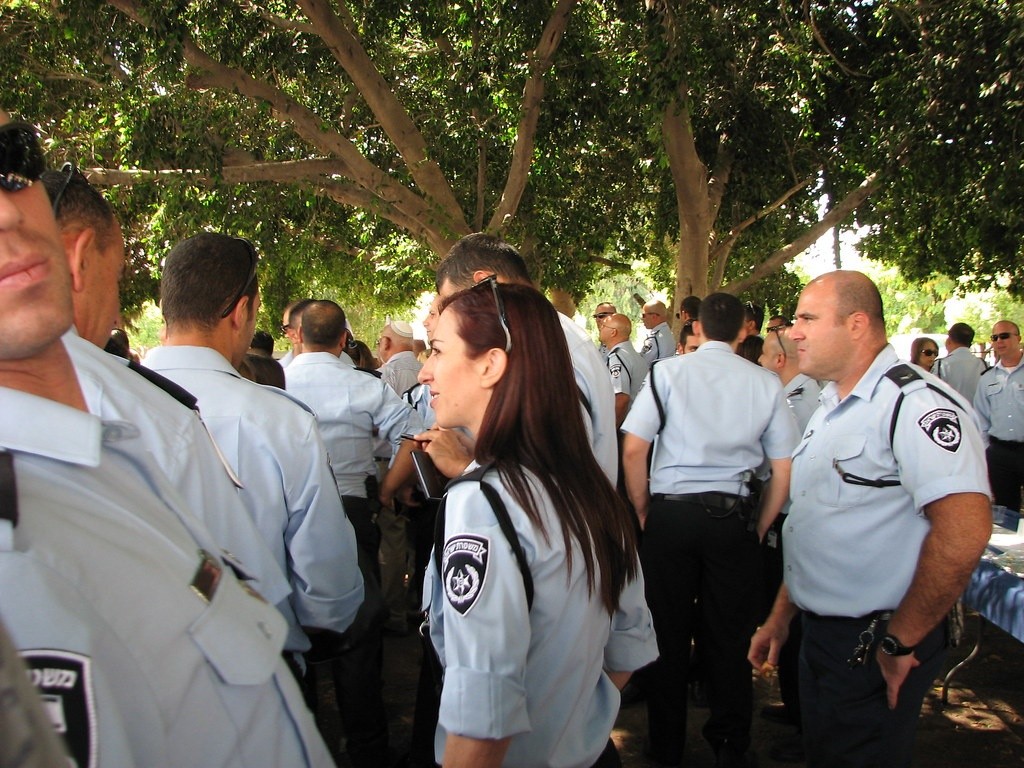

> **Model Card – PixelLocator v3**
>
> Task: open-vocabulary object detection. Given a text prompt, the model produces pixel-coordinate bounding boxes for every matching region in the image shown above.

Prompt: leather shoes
[769,736,806,762]
[762,701,794,723]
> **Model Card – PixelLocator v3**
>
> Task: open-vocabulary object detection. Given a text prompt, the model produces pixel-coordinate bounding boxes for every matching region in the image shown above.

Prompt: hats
[391,320,413,337]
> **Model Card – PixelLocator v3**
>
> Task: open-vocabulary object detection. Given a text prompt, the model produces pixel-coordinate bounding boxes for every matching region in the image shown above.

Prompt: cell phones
[400,433,414,440]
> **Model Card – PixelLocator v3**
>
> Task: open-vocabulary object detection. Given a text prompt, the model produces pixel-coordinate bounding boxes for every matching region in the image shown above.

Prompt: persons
[973,320,1024,512]
[1,108,336,768]
[911,337,939,372]
[104,299,829,621]
[746,270,992,767]
[620,292,802,768]
[39,170,315,690]
[140,232,390,768]
[418,232,676,768]
[930,322,988,409]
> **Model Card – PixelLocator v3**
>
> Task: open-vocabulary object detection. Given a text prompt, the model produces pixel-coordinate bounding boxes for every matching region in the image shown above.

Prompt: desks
[942,503,1024,733]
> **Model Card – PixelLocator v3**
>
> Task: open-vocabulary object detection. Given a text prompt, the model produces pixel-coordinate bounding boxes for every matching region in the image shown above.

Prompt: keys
[847,631,874,669]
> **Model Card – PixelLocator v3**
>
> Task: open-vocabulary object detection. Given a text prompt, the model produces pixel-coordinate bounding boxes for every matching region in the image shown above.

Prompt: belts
[654,491,746,510]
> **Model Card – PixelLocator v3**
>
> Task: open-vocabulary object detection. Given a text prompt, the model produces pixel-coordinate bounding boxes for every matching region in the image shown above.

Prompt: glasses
[478,274,513,353]
[223,237,259,320]
[922,349,939,357]
[53,160,93,223]
[990,333,1020,341]
[746,301,756,315]
[280,324,289,336]
[641,312,661,317]
[766,325,787,332]
[675,312,680,321]
[0,119,45,192]
[684,319,693,327]
[593,312,613,319]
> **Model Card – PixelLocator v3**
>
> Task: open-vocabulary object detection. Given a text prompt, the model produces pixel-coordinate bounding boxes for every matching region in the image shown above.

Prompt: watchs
[880,633,916,656]
